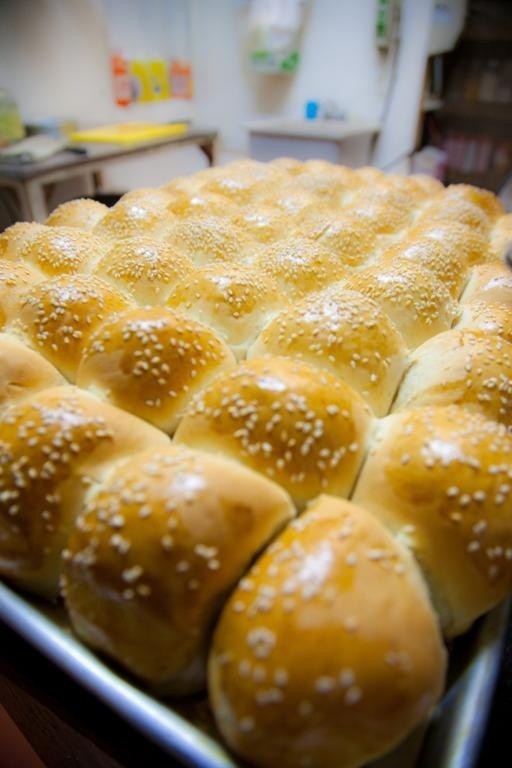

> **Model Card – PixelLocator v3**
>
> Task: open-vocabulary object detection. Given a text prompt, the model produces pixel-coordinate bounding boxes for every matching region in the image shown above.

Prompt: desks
[247,121,380,167]
[0,122,220,223]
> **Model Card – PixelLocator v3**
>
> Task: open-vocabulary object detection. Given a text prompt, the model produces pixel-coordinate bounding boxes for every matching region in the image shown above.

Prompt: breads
[0,156,512,768]
